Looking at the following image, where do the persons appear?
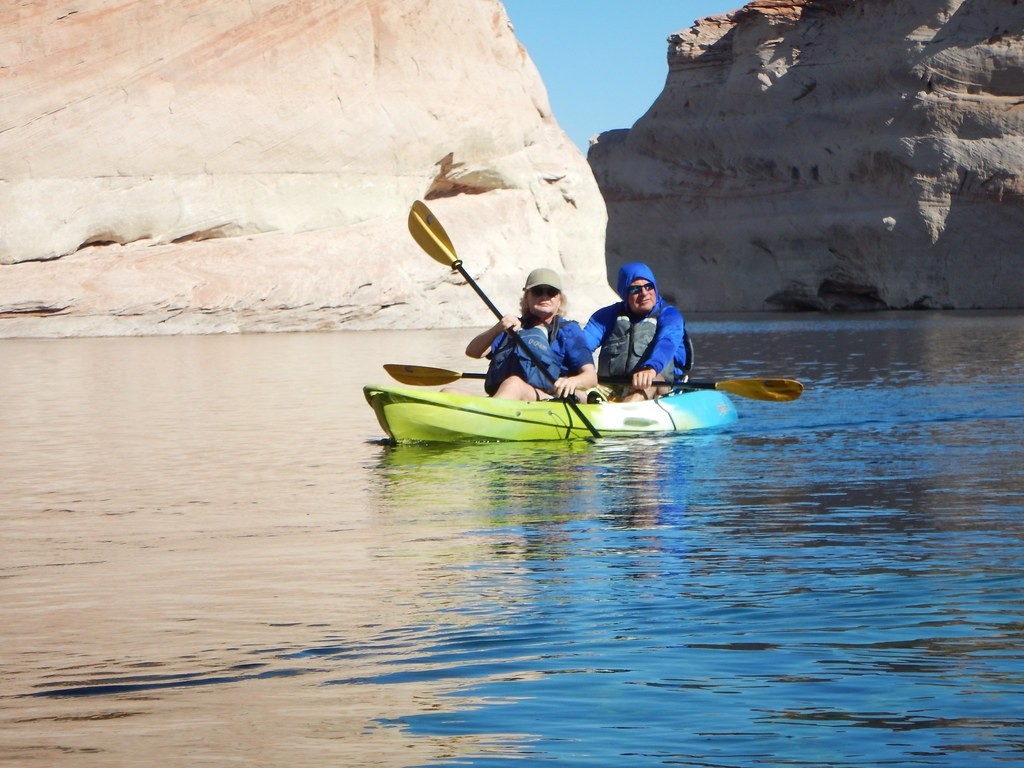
[580,262,688,405]
[466,269,598,402]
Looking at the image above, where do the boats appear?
[363,382,739,443]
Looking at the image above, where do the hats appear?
[524,267,561,295]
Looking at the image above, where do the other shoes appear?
[587,389,607,404]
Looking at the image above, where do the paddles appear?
[407,199,603,441]
[382,357,804,403]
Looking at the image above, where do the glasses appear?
[529,286,558,297]
[629,283,654,293]
